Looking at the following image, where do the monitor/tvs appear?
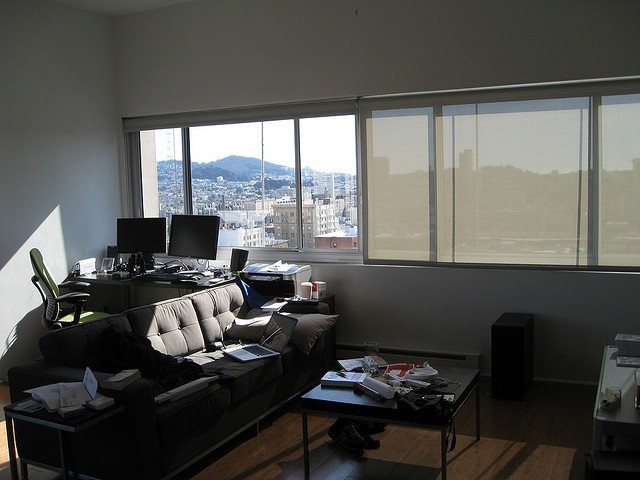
[114,219,167,258]
[167,214,222,273]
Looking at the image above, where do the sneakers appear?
[328,417,366,450]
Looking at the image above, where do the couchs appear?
[7,277,339,477]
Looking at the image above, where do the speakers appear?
[230,248,249,271]
[106,246,118,262]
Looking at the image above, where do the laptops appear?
[223,310,297,364]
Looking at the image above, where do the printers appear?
[240,262,311,300]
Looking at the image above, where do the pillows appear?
[226,307,340,355]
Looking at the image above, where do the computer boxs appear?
[492,312,535,402]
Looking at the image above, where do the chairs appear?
[29,247,119,330]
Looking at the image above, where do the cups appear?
[605,385,621,407]
[300,281,314,300]
[364,340,380,378]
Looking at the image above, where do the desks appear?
[591,344,639,452]
[307,292,337,317]
[72,262,240,309]
[3,393,124,479]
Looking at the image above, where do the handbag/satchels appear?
[397,387,444,420]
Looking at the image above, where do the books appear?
[42,366,114,417]
[610,333,640,367]
[100,369,141,391]
[320,371,367,387]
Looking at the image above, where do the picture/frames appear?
[101,257,115,272]
